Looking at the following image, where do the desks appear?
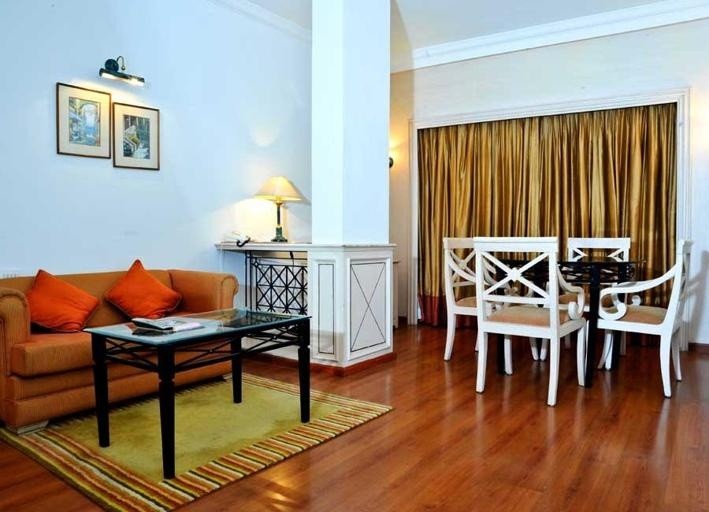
[495,257,646,388]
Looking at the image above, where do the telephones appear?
[221,229,255,248]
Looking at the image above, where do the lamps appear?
[254,176,302,243]
[99,56,145,87]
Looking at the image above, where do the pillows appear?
[103,259,182,320]
[23,269,100,334]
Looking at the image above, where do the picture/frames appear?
[55,82,112,159]
[113,102,161,170]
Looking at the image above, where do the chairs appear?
[564,237,632,355]
[597,238,694,398]
[442,236,539,375]
[473,236,587,406]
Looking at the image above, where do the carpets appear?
[0,368,395,512]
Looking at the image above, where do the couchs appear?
[0,259,239,436]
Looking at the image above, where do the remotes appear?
[131,316,174,333]
